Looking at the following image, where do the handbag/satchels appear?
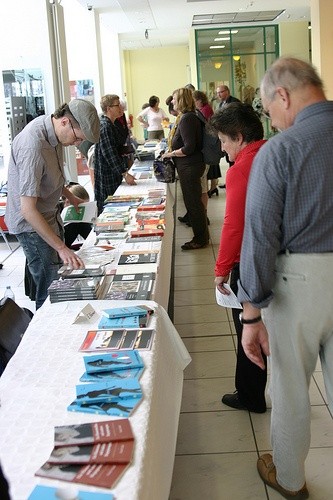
[153,150,175,183]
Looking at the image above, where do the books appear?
[98,314,149,328]
[102,304,154,319]
[78,329,155,350]
[68,351,145,417]
[95,188,166,241]
[132,143,157,178]
[28,419,134,500]
[47,247,157,299]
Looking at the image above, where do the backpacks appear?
[0,297,32,365]
[179,108,224,166]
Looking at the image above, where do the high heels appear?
[207,187,219,198]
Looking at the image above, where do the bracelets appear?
[172,150,176,156]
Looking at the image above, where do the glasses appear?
[69,119,83,143]
[110,103,122,107]
[216,90,227,95]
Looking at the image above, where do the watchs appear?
[239,313,261,324]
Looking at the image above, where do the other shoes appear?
[177,216,191,226]
[219,184,226,189]
[181,239,208,250]
[257,453,309,500]
[222,391,267,413]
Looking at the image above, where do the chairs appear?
[0,297,31,355]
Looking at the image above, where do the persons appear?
[211,102,270,412]
[163,89,210,249]
[216,86,241,188]
[237,58,333,500]
[24,183,89,301]
[166,84,218,225]
[94,93,137,218]
[136,96,169,140]
[5,98,101,309]
[193,91,222,196]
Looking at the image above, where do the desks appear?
[0,143,194,500]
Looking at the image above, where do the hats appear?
[69,98,101,143]
